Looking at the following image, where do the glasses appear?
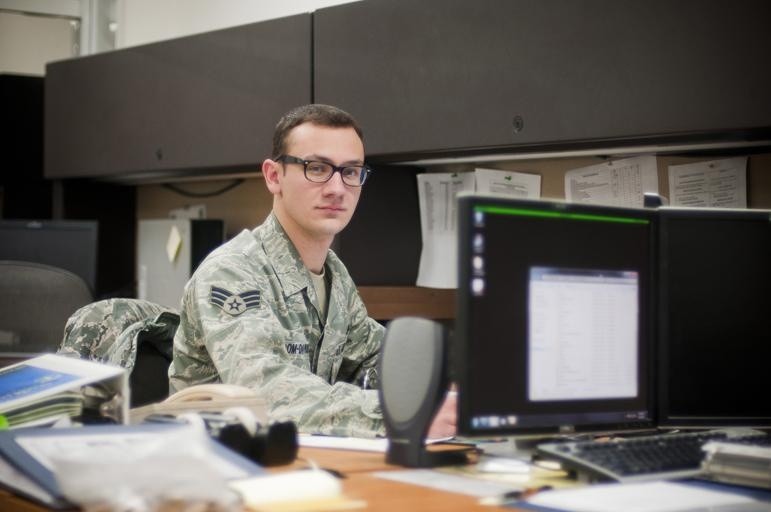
[271,153,371,188]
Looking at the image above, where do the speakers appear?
[377,318,469,467]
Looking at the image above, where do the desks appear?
[0,442,771,512]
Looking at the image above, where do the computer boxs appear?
[137,220,227,313]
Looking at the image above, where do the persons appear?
[166,103,503,442]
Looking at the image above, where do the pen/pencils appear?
[505,484,553,501]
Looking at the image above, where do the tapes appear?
[221,407,260,436]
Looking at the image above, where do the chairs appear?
[0,260,95,352]
[64,296,179,409]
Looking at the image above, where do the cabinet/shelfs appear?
[314,1,770,169]
[43,10,312,179]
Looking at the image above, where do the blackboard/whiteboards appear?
[337,169,438,287]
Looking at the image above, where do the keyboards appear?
[534,432,770,485]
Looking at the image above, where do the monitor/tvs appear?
[658,206,771,425]
[453,191,659,449]
